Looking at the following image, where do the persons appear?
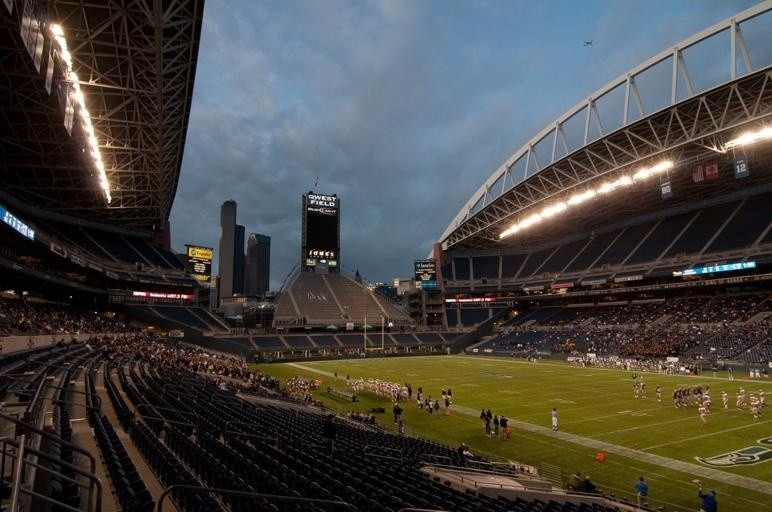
[487,409,493,420]
[494,414,499,437]
[551,407,559,433]
[480,409,487,425]
[484,415,492,438]
[462,446,476,460]
[458,441,466,457]
[630,380,771,429]
[569,471,582,488]
[500,415,509,438]
[634,475,649,507]
[695,480,718,512]
[578,475,597,493]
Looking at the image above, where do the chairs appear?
[272,404,444,511]
[445,445,604,510]
[1,372,270,511]
